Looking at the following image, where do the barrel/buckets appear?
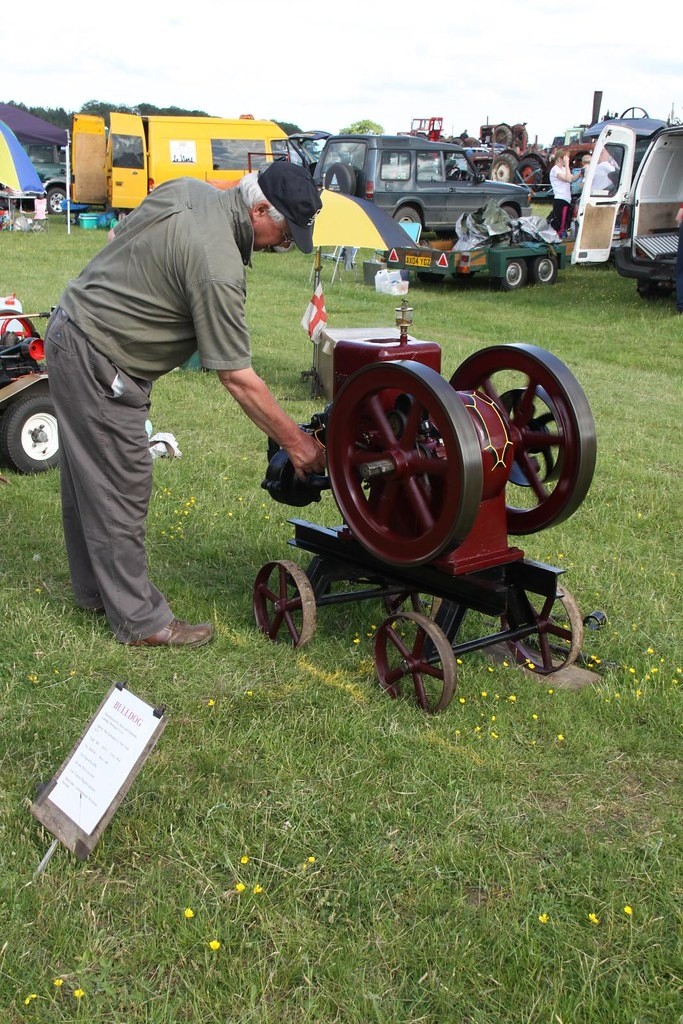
[362,262,381,286]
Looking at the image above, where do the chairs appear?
[21,199,50,234]
[309,245,358,285]
[419,158,440,181]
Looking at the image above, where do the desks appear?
[0,196,37,232]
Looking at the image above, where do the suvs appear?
[312,134,533,238]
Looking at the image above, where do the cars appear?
[21,144,67,214]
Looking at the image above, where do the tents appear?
[0,102,70,234]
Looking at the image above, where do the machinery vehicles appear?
[393,117,597,189]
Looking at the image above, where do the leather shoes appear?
[129,615,213,650]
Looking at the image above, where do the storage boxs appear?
[382,281,410,295]
[78,216,98,229]
[318,327,415,402]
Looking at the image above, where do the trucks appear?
[572,123,683,300]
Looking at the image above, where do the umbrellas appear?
[0,117,47,193]
[314,186,418,332]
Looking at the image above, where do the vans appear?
[446,148,490,171]
[72,112,289,209]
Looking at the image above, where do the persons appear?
[580,148,617,193]
[675,202,683,311]
[460,130,468,142]
[550,149,580,237]
[41,158,327,647]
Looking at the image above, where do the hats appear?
[258,162,325,253]
